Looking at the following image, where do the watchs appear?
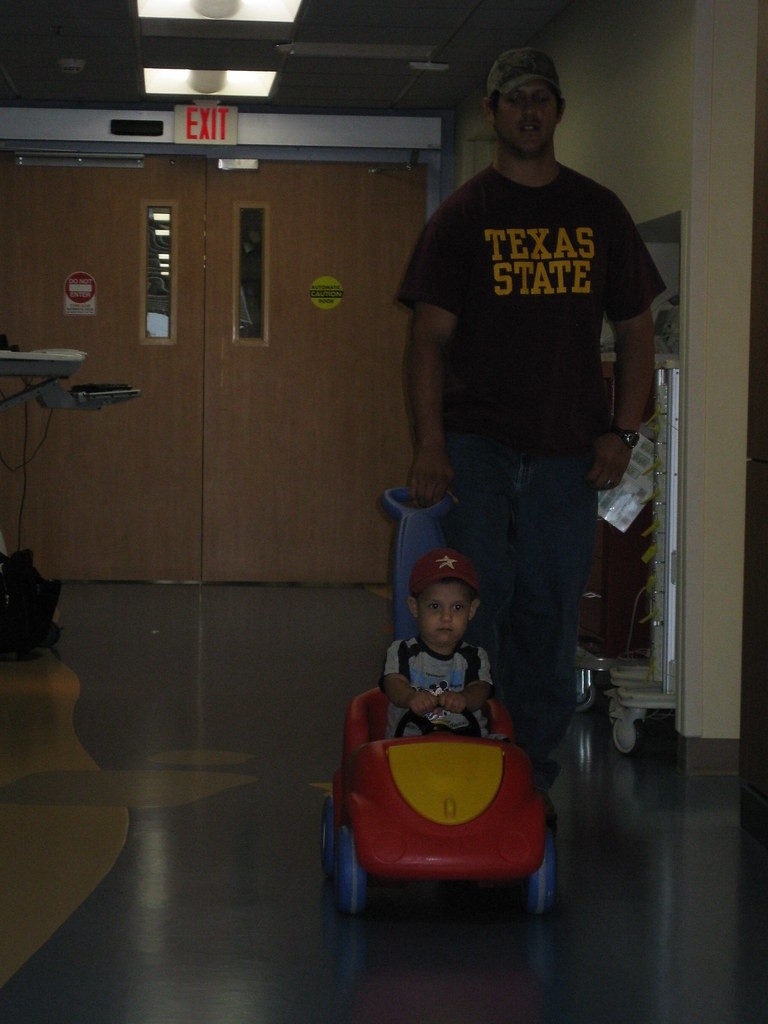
[608,424,640,451]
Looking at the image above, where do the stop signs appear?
[65,270,97,305]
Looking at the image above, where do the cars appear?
[321,688,560,922]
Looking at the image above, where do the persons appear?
[378,547,500,740]
[406,50,656,837]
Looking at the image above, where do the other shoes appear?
[535,788,558,821]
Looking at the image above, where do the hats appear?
[487,47,561,102]
[408,548,481,592]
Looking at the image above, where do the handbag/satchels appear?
[0,548,63,659]
